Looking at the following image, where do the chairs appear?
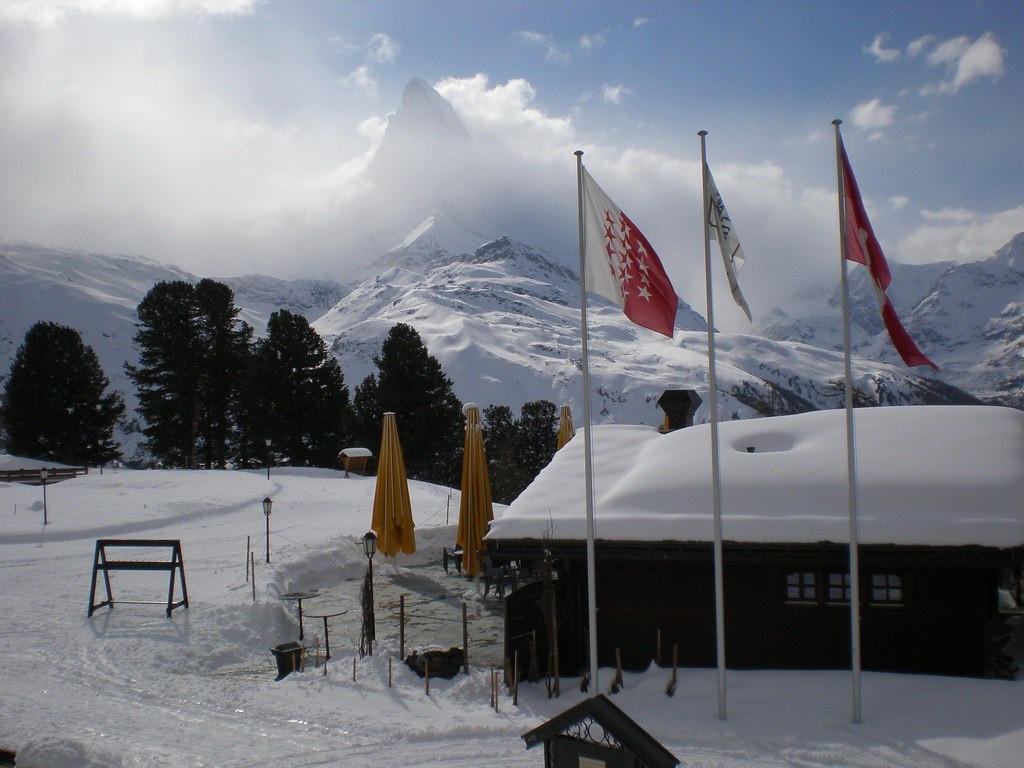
[443,543,464,576]
[475,550,518,601]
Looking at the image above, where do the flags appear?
[581,165,678,340]
[706,163,752,324]
[839,133,941,373]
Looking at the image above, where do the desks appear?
[303,607,348,660]
[279,589,321,641]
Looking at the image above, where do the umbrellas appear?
[456,405,494,592]
[371,411,416,568]
[558,404,576,451]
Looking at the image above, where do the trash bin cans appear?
[268,641,303,682]
